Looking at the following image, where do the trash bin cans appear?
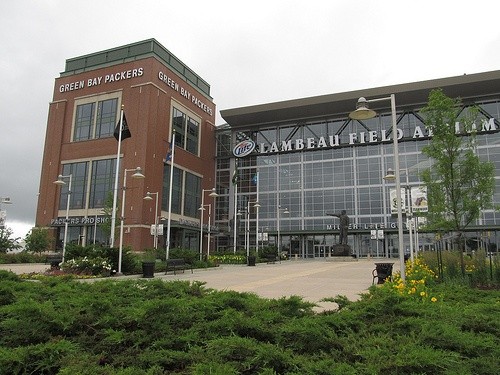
[247,256,256,265]
[374,262,394,284]
[141,260,156,278]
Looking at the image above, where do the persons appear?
[326,210,349,245]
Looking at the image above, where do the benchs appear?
[165,259,194,275]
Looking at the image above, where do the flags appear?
[113,112,131,142]
[165,140,175,161]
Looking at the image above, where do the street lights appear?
[197,204,211,256]
[246,201,262,261]
[349,94,406,285]
[142,191,158,248]
[276,206,290,255]
[200,187,219,258]
[236,207,246,250]
[52,173,72,263]
